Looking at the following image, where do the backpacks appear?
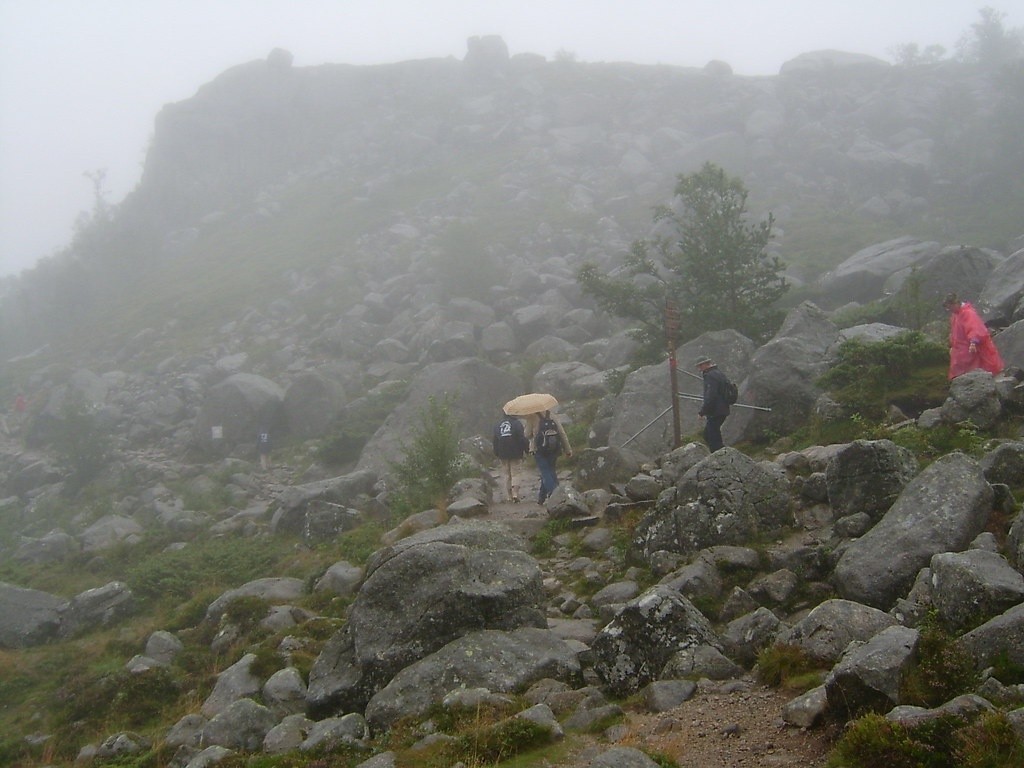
[535,409,563,454]
[710,369,738,404]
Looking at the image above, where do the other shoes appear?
[512,493,520,503]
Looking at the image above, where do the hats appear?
[694,356,711,366]
[943,292,959,306]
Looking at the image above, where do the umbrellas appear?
[502,393,559,416]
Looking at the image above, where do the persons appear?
[493,414,529,502]
[695,354,732,452]
[942,293,1003,380]
[257,434,272,470]
[524,409,573,505]
